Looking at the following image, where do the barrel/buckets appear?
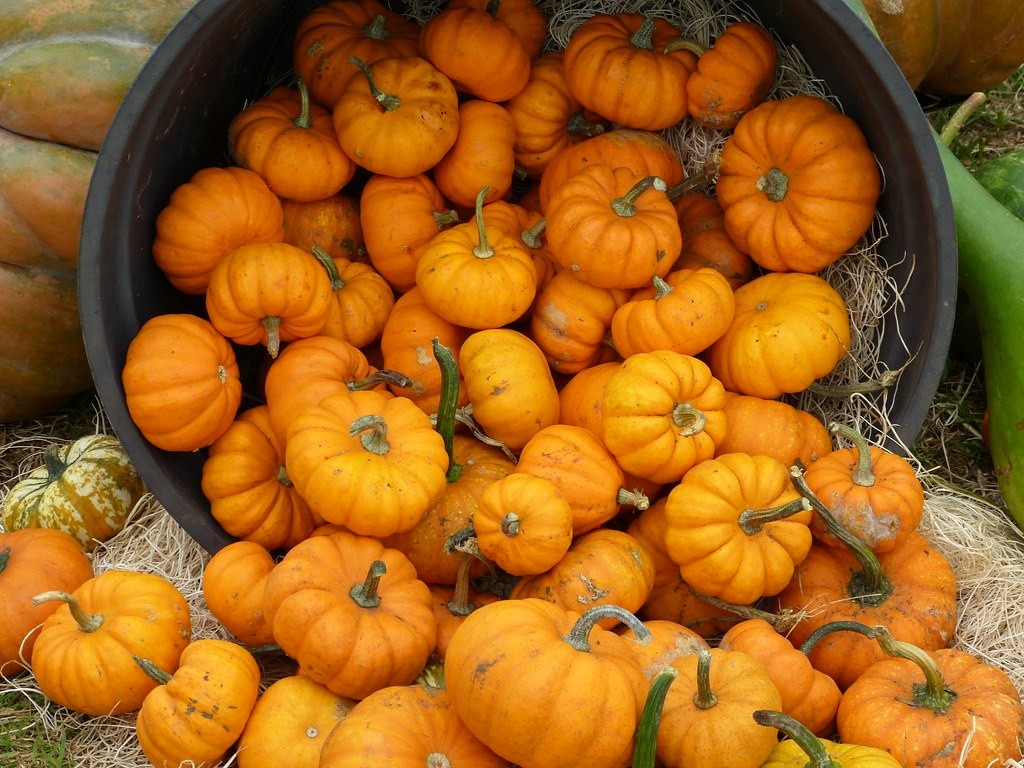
[76,0,956,557]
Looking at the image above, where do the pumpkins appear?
[0,1,1024,768]
